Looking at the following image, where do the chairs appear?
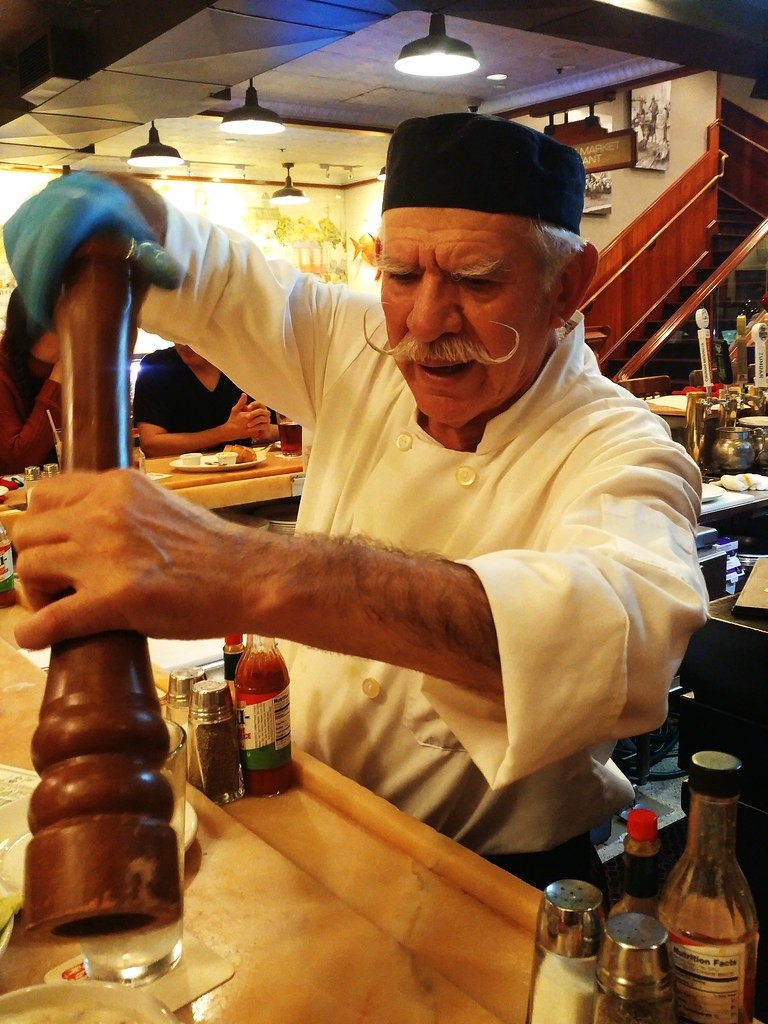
[618,375,672,400]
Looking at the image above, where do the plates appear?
[219,504,300,536]
[0,981,182,1024]
[0,790,198,893]
[169,453,267,472]
[701,483,726,504]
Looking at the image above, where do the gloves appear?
[6,170,183,335]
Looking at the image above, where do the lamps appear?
[270,163,304,198]
[377,167,386,180]
[48,164,72,183]
[221,79,285,134]
[393,13,482,77]
[128,119,184,168]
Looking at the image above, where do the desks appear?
[643,394,752,429]
[695,467,768,525]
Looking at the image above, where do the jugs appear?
[712,427,768,474]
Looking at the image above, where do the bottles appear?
[187,680,246,805]
[166,667,207,735]
[24,466,41,492]
[659,751,759,1024]
[130,428,146,476]
[591,912,677,1024]
[0,521,16,609]
[222,633,246,706]
[604,809,660,917]
[42,464,60,478]
[530,879,604,1023]
[235,632,294,796]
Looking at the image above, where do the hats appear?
[382,113,584,235]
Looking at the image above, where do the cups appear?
[52,429,62,473]
[82,716,188,988]
[216,452,237,466]
[179,453,202,466]
[276,412,302,457]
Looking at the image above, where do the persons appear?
[132,342,280,459]
[0,287,65,477]
[11,114,709,918]
